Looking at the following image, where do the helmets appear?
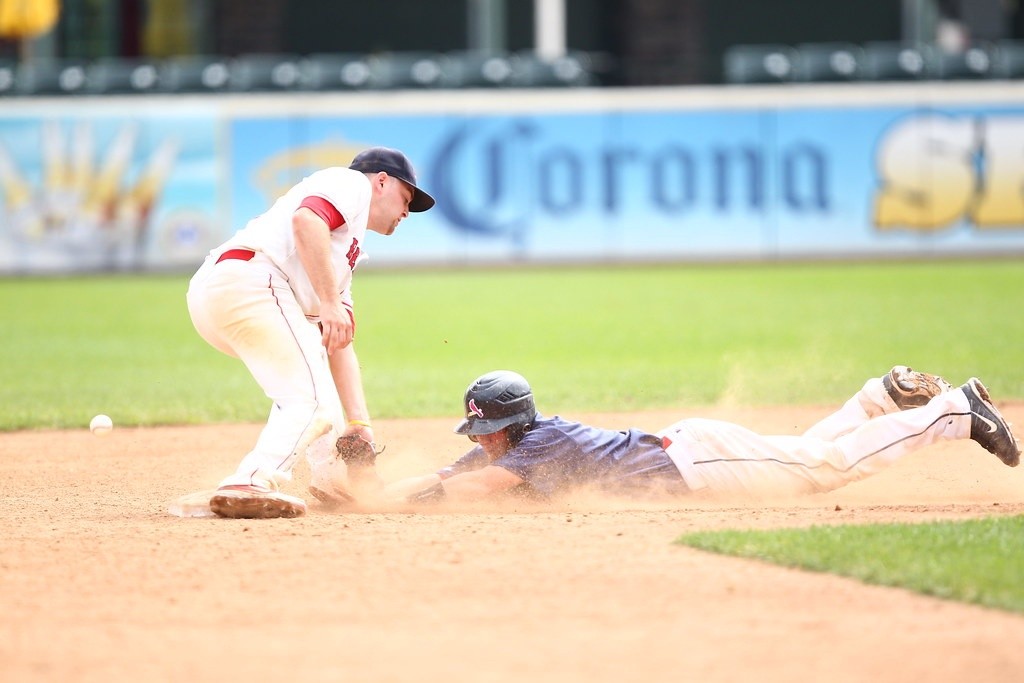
[455,371,540,438]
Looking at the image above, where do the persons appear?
[187,147,435,519]
[309,365,1021,513]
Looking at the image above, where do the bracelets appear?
[348,420,371,427]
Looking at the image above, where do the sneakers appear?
[883,364,954,410]
[961,375,1022,469]
[209,480,306,521]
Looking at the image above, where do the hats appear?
[345,146,435,213]
